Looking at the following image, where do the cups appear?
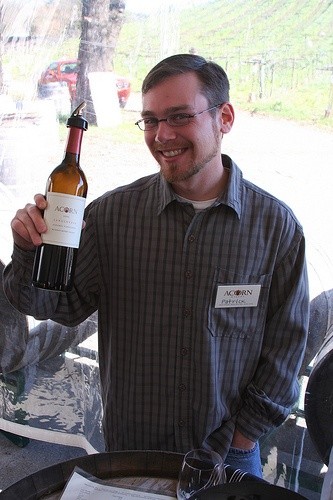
[175,449,226,500]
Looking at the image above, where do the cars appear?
[36,58,132,109]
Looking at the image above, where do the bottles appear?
[31,103,89,293]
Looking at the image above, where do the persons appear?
[0,261,99,448]
[3,54,310,479]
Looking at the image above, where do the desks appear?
[0,451,309,500]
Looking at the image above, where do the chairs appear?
[0,319,98,449]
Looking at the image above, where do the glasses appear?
[135,103,223,131]
